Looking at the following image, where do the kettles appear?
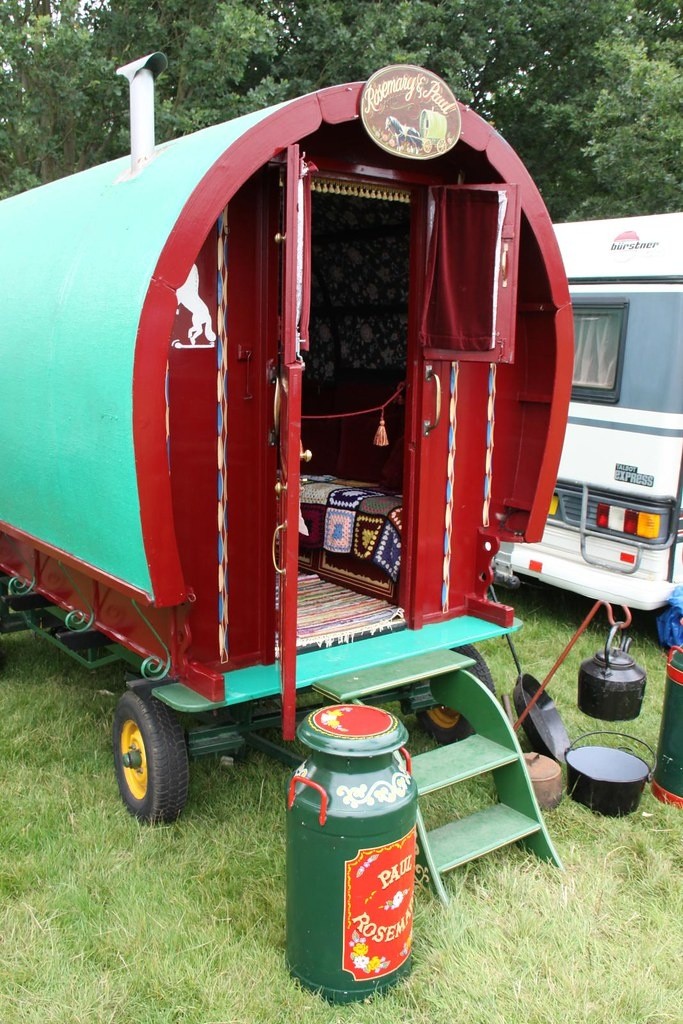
[579,622,647,721]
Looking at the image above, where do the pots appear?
[506,633,570,763]
[523,752,564,810]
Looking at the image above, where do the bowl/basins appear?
[564,731,657,817]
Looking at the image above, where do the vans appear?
[489,210,683,612]
